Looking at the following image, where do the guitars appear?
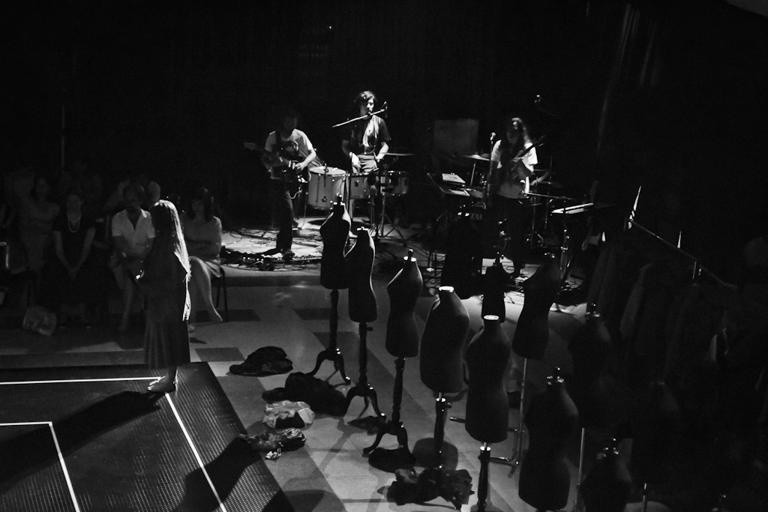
[491,134,545,191]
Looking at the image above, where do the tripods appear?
[426,209,445,278]
[375,195,409,250]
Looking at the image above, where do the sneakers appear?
[282,250,296,262]
[146,375,176,393]
[207,303,222,322]
[115,318,131,332]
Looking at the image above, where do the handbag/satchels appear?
[22,304,58,336]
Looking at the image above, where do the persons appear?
[1,159,224,339]
[310,193,632,511]
[261,108,318,261]
[484,115,539,281]
[336,89,393,223]
[134,198,191,395]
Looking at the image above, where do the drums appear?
[349,172,377,199]
[377,169,412,197]
[308,167,346,210]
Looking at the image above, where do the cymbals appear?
[386,153,411,157]
[455,155,490,164]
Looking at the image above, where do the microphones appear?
[382,97,391,122]
[529,133,549,149]
